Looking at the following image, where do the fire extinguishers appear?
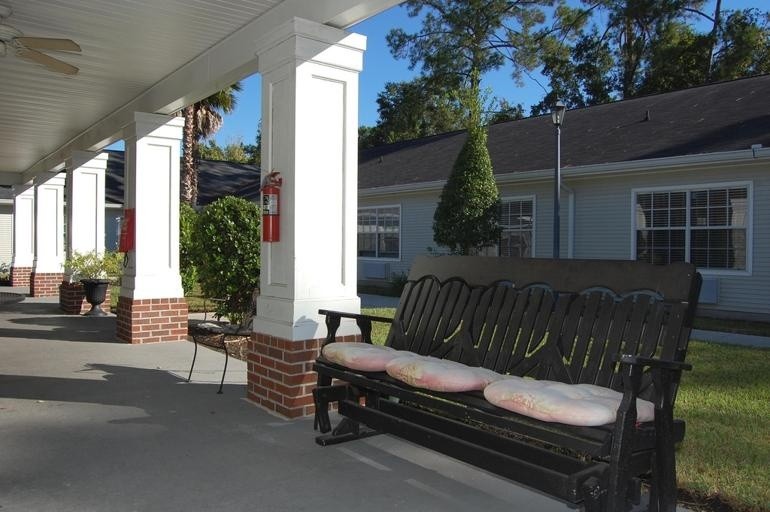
[118,217,128,254]
[259,172,282,242]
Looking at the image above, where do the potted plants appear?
[61,250,121,316]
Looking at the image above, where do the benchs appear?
[311,253,704,512]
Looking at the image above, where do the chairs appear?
[186,283,259,395]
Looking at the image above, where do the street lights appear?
[549,92,568,258]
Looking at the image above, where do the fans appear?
[0,3,83,77]
